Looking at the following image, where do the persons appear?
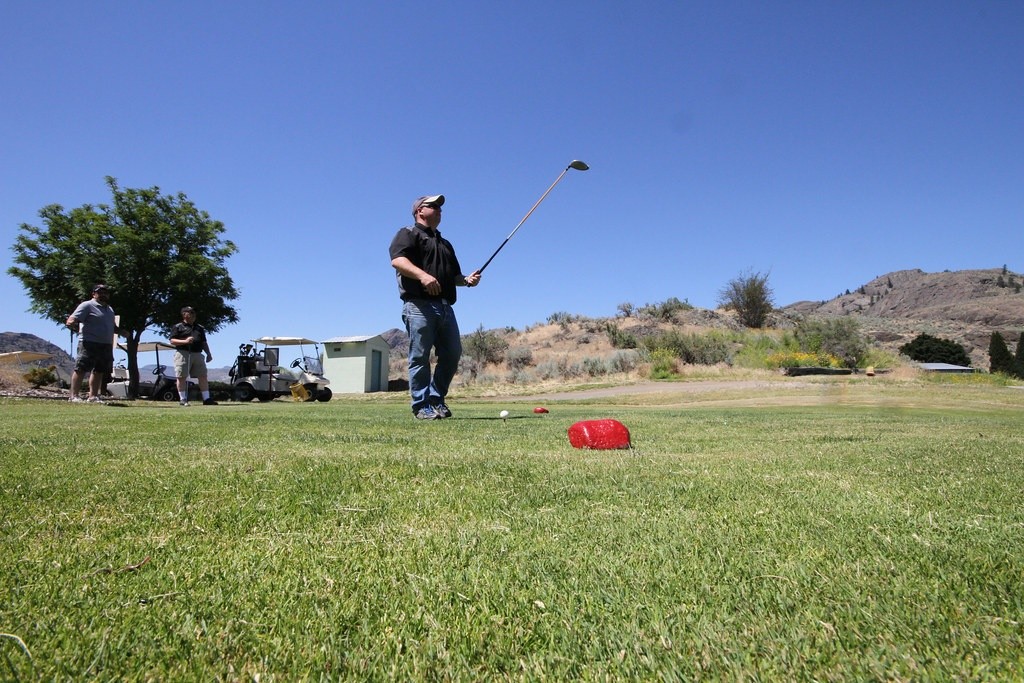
[66,284,115,404]
[169,306,219,407]
[388,195,482,421]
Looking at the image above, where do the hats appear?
[411,194,445,215]
[181,306,194,312]
[92,284,108,292]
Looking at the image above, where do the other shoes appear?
[68,396,85,403]
[202,398,217,405]
[180,399,188,406]
[87,397,106,404]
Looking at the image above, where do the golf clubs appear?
[465,157,592,289]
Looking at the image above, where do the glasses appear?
[420,204,440,210]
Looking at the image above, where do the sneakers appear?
[416,405,441,421]
[430,402,451,418]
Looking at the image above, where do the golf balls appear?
[499,409,510,420]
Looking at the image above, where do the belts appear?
[404,298,450,306]
